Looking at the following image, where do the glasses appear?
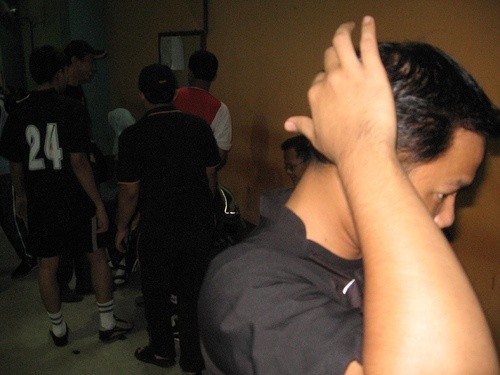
[284,159,306,171]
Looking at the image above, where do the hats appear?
[63,40,108,58]
[138,63,180,93]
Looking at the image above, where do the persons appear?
[115,64,218,375]
[0,69,38,279]
[169,51,235,183]
[56,39,98,306]
[282,133,330,186]
[195,16,500,375]
[0,44,137,348]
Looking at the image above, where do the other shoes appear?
[59,287,83,303]
[134,346,175,368]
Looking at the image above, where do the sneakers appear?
[49,321,69,348]
[11,254,39,280]
[99,314,135,344]
